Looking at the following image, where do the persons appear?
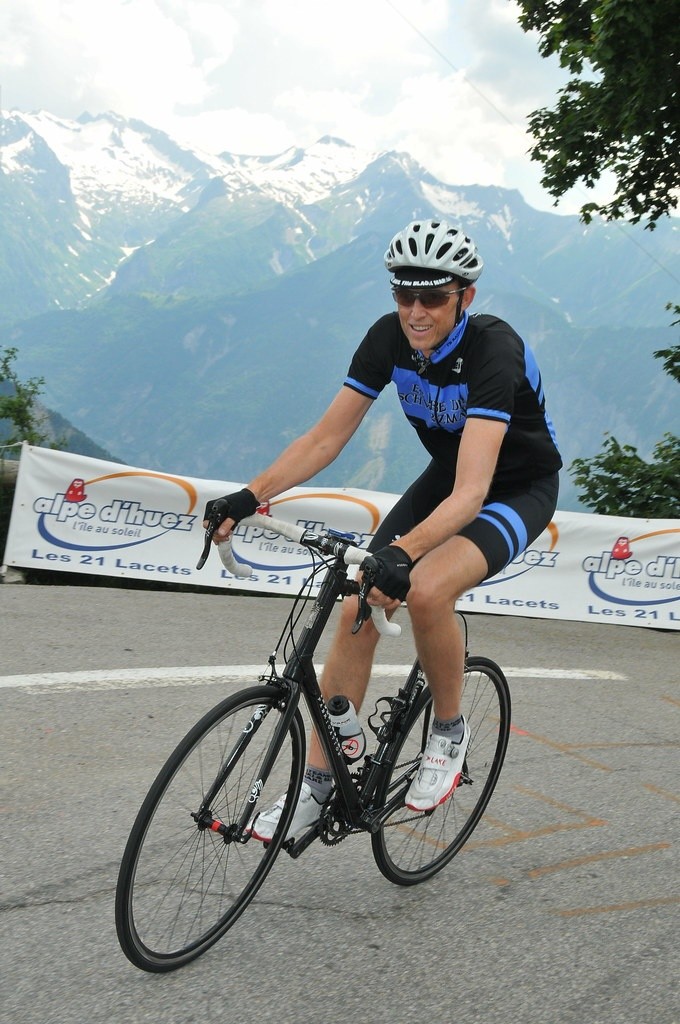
[202,220,562,841]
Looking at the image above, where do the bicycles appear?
[114,499,512,974]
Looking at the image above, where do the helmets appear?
[384,218,484,289]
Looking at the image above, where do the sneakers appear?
[245,777,336,844]
[405,715,471,812]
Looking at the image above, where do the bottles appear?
[327,696,365,759]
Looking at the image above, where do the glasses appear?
[390,285,467,309]
